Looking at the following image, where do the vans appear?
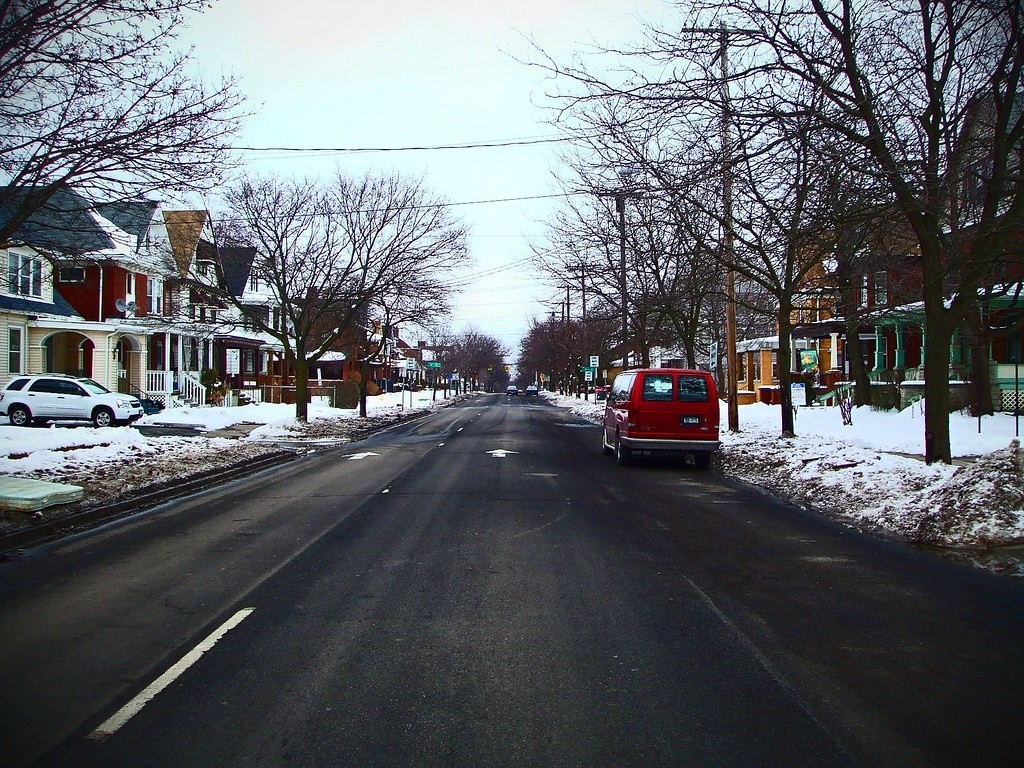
[596,368,721,470]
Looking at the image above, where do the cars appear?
[506,385,518,396]
[526,386,538,395]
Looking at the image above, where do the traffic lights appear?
[505,366,509,372]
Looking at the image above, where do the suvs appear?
[0,373,145,428]
